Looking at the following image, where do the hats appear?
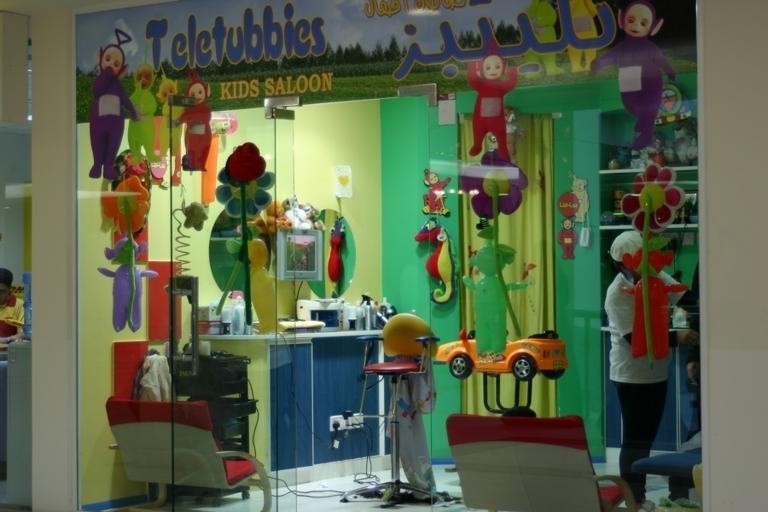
[610,231,642,262]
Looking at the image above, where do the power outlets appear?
[329,412,363,432]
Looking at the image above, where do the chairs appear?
[104,395,275,512]
[348,312,439,507]
[444,411,641,512]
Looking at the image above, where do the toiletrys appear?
[231,296,245,335]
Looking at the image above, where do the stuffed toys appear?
[592,0,678,148]
[97,234,158,332]
[89,27,139,181]
[303,203,326,231]
[155,64,182,157]
[129,43,159,162]
[416,216,453,304]
[422,169,451,215]
[620,246,687,361]
[665,118,698,165]
[328,218,345,281]
[266,201,292,232]
[571,175,589,223]
[557,219,577,258]
[178,70,213,172]
[461,243,528,356]
[282,192,312,231]
[465,38,518,158]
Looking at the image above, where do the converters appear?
[343,409,354,418]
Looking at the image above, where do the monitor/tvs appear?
[277,229,325,282]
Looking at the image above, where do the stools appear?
[631,446,702,502]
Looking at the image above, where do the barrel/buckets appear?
[22,272,32,340]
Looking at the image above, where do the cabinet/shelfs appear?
[602,324,699,478]
[168,351,250,508]
[600,165,698,232]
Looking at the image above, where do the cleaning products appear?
[360,295,373,329]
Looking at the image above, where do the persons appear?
[685,314,700,384]
[0,268,24,361]
[604,230,697,512]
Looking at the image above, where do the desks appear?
[188,327,388,488]
